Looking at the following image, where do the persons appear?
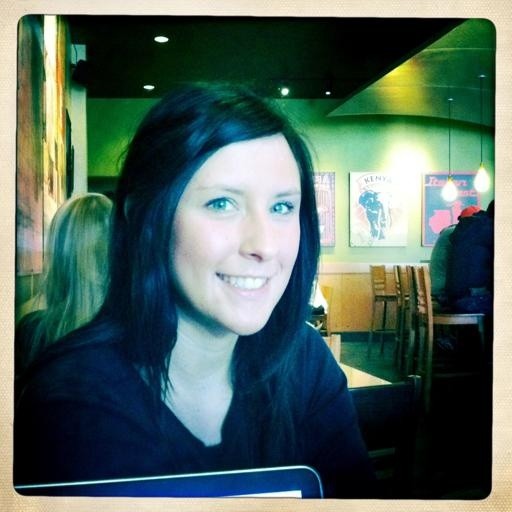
[426,205,482,362]
[446,193,493,350]
[14,76,389,498]
[14,187,117,370]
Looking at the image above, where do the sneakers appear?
[436,335,456,351]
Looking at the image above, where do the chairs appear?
[322,334,341,362]
[367,261,485,408]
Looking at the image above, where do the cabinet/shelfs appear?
[421,170,482,247]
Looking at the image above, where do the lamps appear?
[473,75,491,194]
[441,99,459,203]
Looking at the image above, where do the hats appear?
[461,206,480,218]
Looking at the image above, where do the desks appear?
[336,363,392,389]
